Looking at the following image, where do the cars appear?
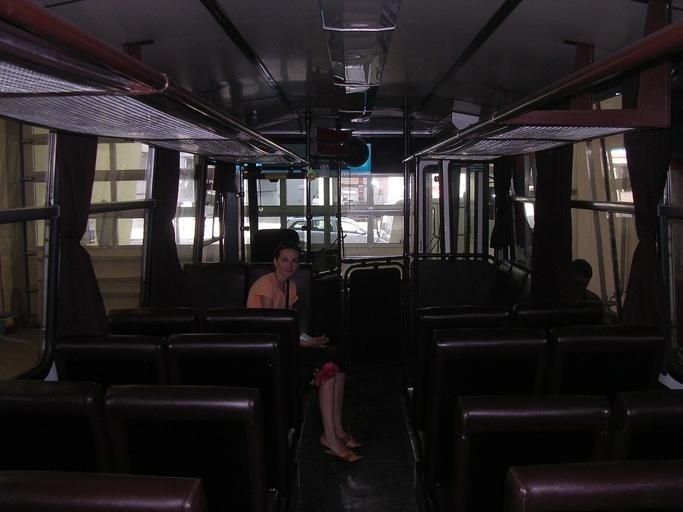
[282,218,387,245]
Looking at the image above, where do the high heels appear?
[339,435,363,448]
[322,449,362,462]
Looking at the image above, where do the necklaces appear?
[274,273,285,294]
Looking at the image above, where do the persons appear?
[246,242,365,461]
